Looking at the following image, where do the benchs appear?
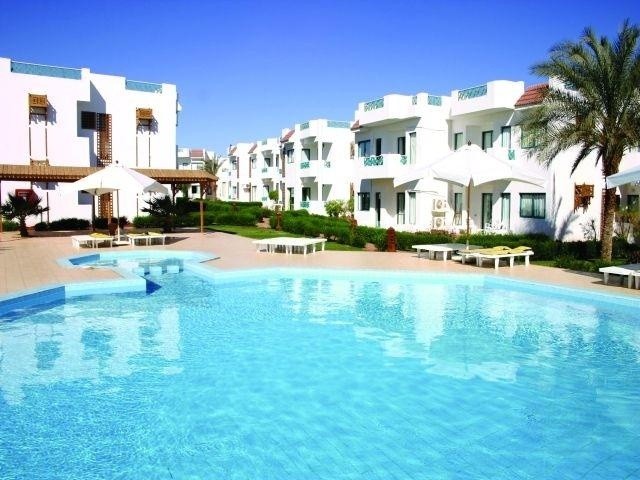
[253,236,327,256]
[596,260,640,291]
[410,242,536,271]
[68,231,166,248]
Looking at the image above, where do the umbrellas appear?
[393,141,545,251]
[69,160,169,242]
[607,165,639,191]
